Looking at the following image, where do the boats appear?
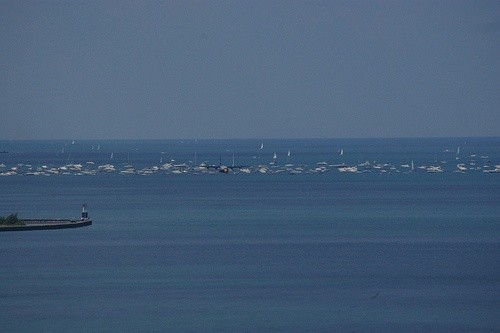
[1,147,498,184]
